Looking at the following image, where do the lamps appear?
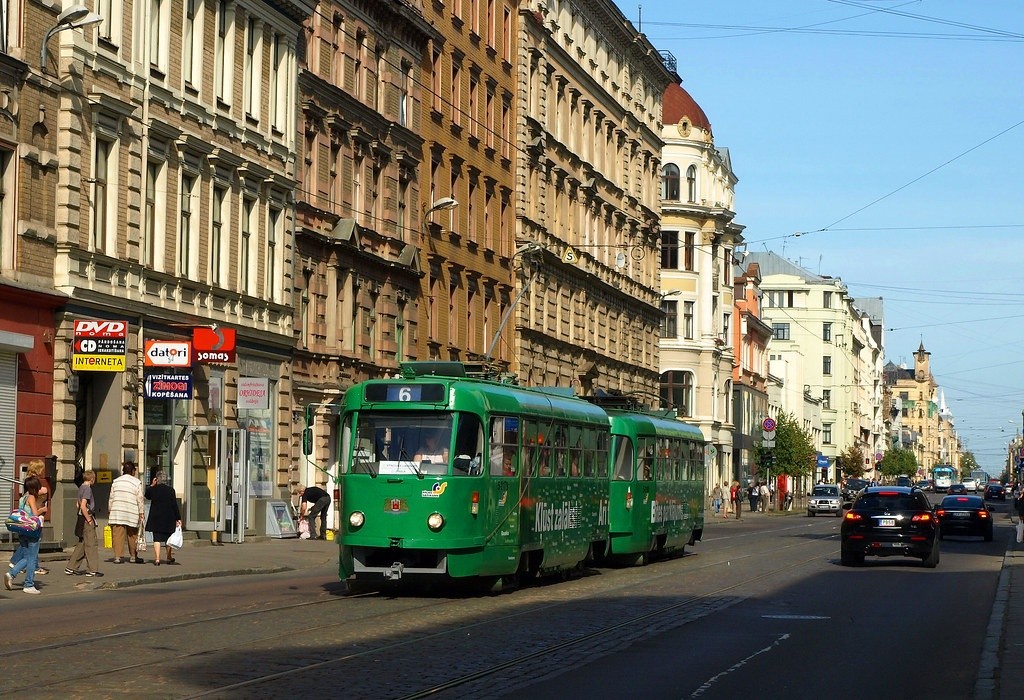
[512,241,541,261]
[424,197,459,221]
[659,291,683,307]
[40,5,104,72]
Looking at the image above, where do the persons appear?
[711,481,773,520]
[108,461,145,564]
[296,484,331,541]
[3,476,47,594]
[65,470,104,577]
[504,454,514,476]
[8,459,49,575]
[412,433,448,465]
[145,471,182,566]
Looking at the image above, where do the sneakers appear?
[23,586,41,594]
[4,573,14,591]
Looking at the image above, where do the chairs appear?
[947,500,955,506]
[830,489,835,494]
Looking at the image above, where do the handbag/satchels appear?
[1016,519,1024,543]
[5,509,42,540]
[75,513,86,538]
[166,524,184,550]
[103,523,113,549]
[136,519,147,551]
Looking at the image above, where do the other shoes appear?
[86,571,104,577]
[154,559,160,566]
[8,562,24,574]
[309,535,317,539]
[129,556,143,563]
[34,567,49,574]
[167,558,175,564]
[113,557,124,564]
[64,567,82,575]
[318,536,326,539]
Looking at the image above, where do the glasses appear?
[133,468,136,471]
[424,436,435,439]
